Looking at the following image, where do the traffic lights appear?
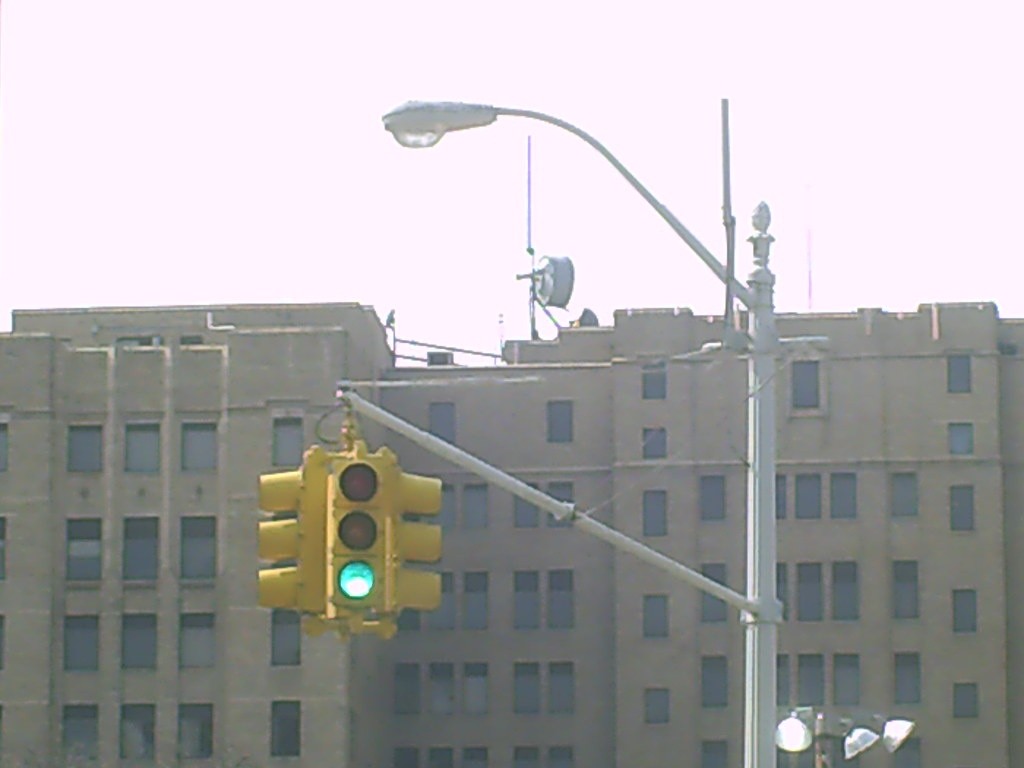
[377,446,442,624]
[255,445,325,622]
[327,452,382,614]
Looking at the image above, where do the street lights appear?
[381,98,781,767]
